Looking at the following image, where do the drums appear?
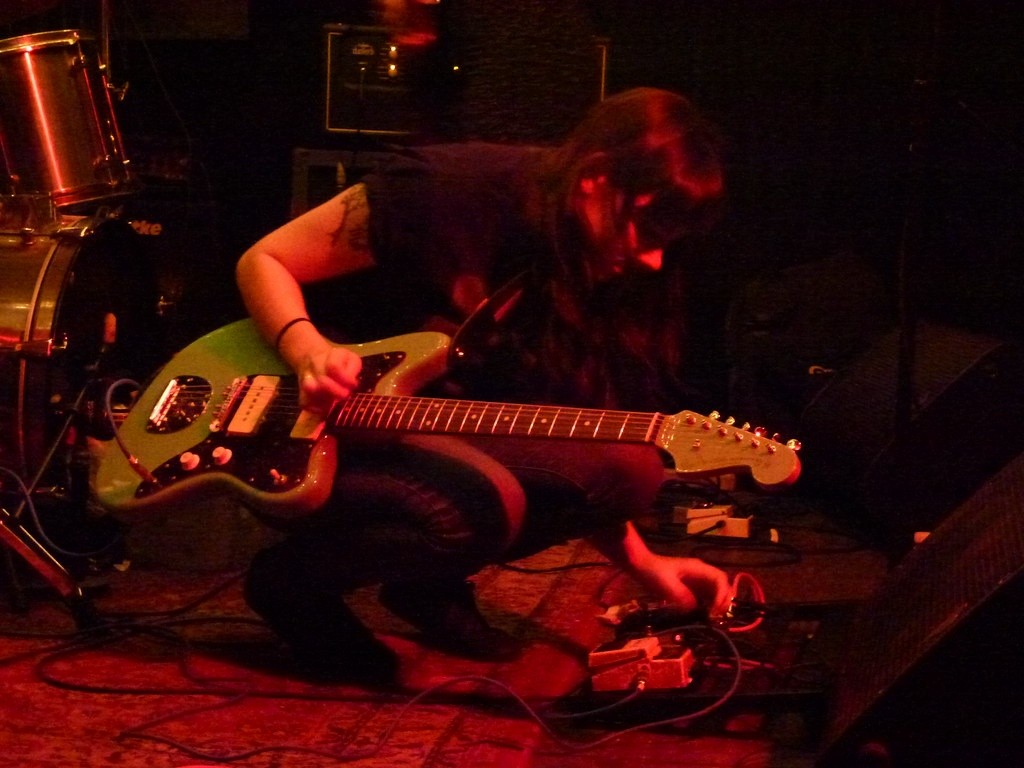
[0,198,112,359]
[0,27,136,211]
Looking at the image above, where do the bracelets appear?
[275,318,313,362]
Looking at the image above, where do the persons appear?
[236,88,734,678]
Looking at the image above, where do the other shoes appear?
[377,576,519,662]
[243,542,398,683]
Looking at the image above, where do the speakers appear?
[795,317,1024,549]
[824,450,1024,768]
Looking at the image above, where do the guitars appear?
[85,311,808,520]
